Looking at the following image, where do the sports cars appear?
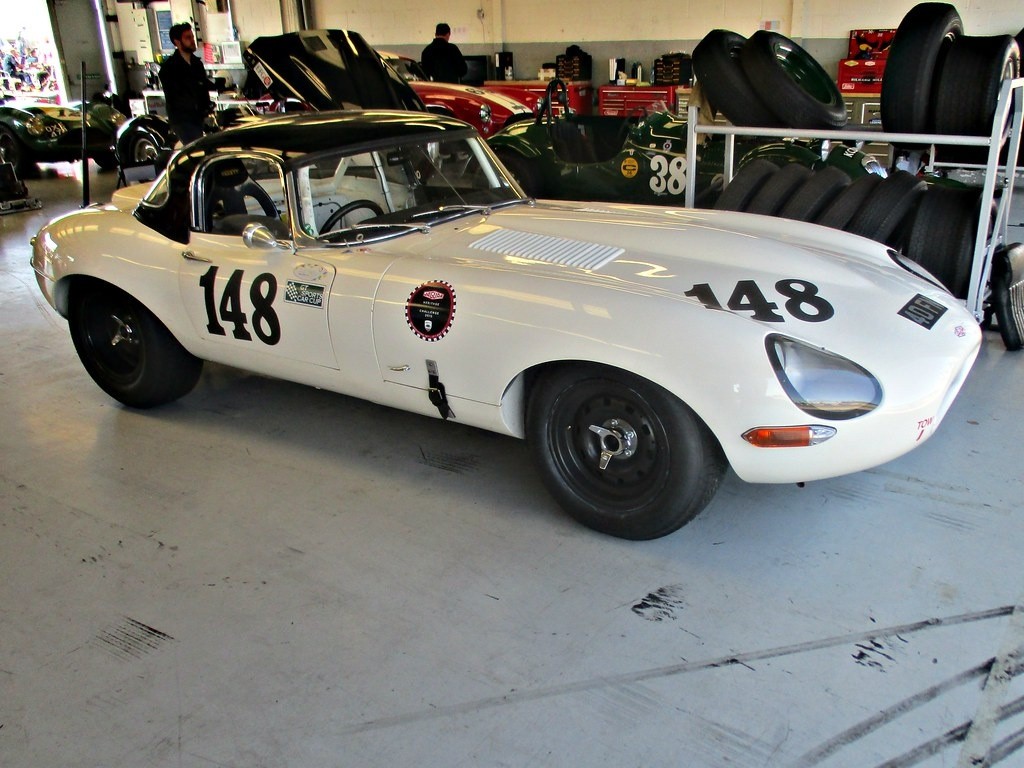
[112,48,544,163]
[31,107,984,540]
[1,98,130,176]
[246,29,890,210]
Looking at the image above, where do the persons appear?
[160,23,216,146]
[421,23,468,84]
[143,61,161,90]
[0,48,50,91]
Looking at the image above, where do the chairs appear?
[197,155,279,233]
